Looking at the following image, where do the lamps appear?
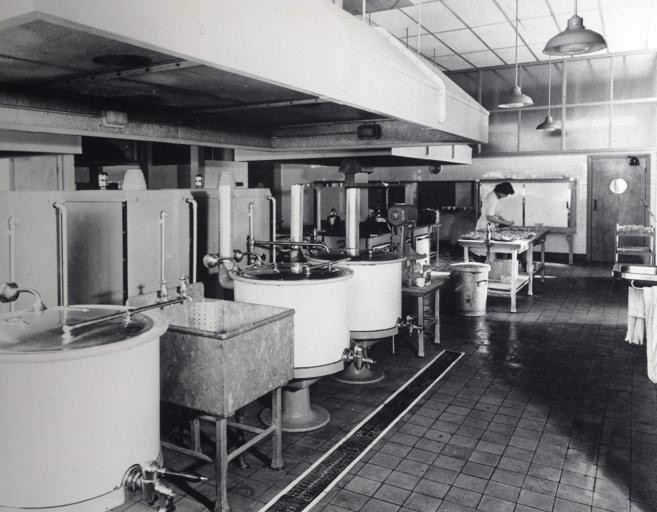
[495,0,607,134]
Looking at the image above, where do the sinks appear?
[127,281,295,419]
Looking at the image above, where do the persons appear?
[469,182,514,262]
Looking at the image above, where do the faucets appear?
[157,281,172,304]
[179,274,193,301]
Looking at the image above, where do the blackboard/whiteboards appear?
[478,177,577,233]
